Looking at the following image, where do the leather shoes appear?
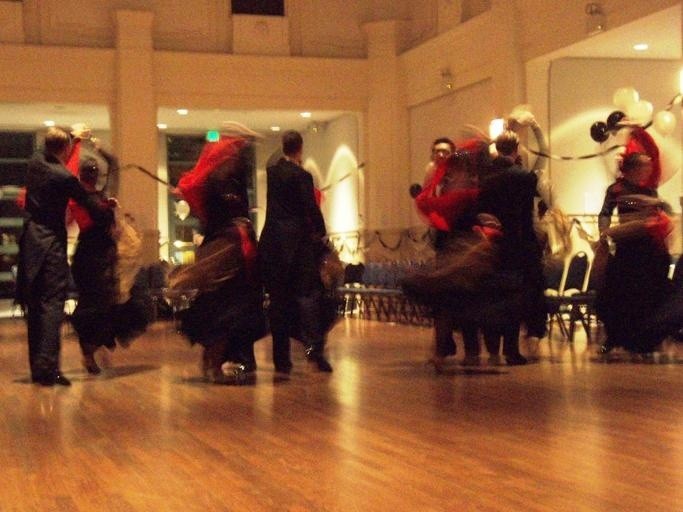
[274,358,293,371]
[505,350,527,364]
[488,355,501,364]
[597,336,614,353]
[461,354,479,365]
[306,344,333,373]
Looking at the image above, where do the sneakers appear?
[31,370,70,386]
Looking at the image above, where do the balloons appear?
[176,200,190,222]
[613,86,638,112]
[628,100,653,127]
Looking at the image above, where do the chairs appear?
[336,259,426,325]
[546,251,600,344]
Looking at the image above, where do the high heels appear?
[235,362,257,374]
[83,358,100,373]
[425,353,456,375]
[208,367,223,383]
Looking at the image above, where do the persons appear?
[11,124,122,386]
[168,123,259,383]
[256,128,344,383]
[398,112,677,365]
[264,154,339,363]
[59,133,163,375]
